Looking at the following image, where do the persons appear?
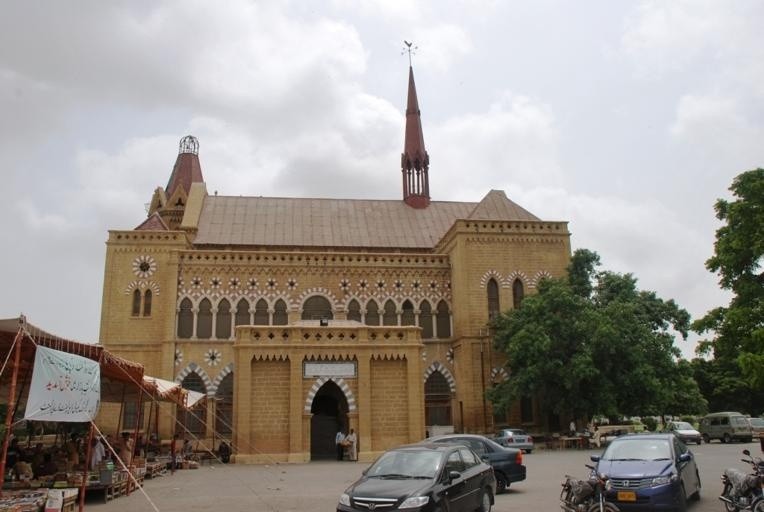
[345,428,357,460]
[589,427,601,449]
[583,426,588,433]
[334,429,346,461]
[643,427,650,432]
[569,420,576,435]
[0,430,232,481]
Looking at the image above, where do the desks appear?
[559,437,582,451]
